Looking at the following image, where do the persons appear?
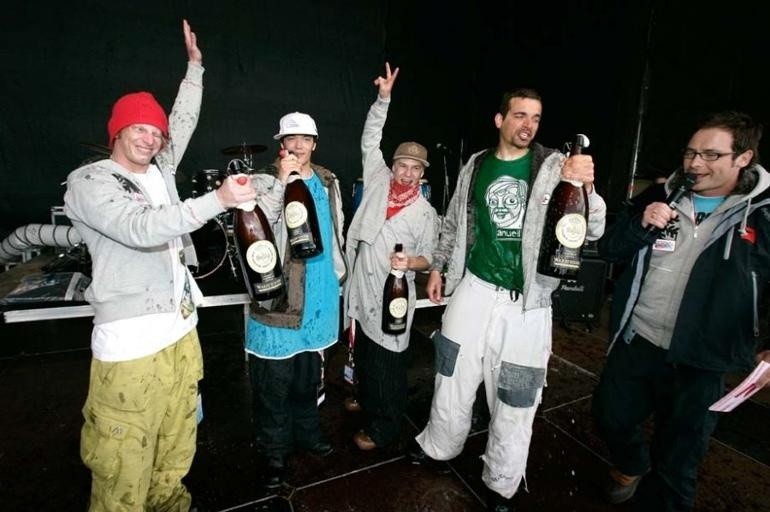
[241,111,350,487]
[592,113,770,512]
[403,89,607,503]
[342,61,441,451]
[62,18,259,512]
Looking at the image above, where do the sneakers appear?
[599,467,643,504]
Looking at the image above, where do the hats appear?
[393,141,430,169]
[107,90,169,150]
[273,110,319,140]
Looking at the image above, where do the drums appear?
[191,169,227,197]
[188,215,229,280]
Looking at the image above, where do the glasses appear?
[682,150,739,161]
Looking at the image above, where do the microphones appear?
[640,171,697,244]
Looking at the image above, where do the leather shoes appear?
[353,430,376,450]
[302,439,334,458]
[345,396,362,413]
[259,468,288,489]
[404,445,437,467]
[490,485,525,512]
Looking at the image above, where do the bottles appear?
[536,139,590,280]
[283,149,325,260]
[381,242,410,337]
[227,159,286,302]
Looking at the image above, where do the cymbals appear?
[223,144,269,155]
